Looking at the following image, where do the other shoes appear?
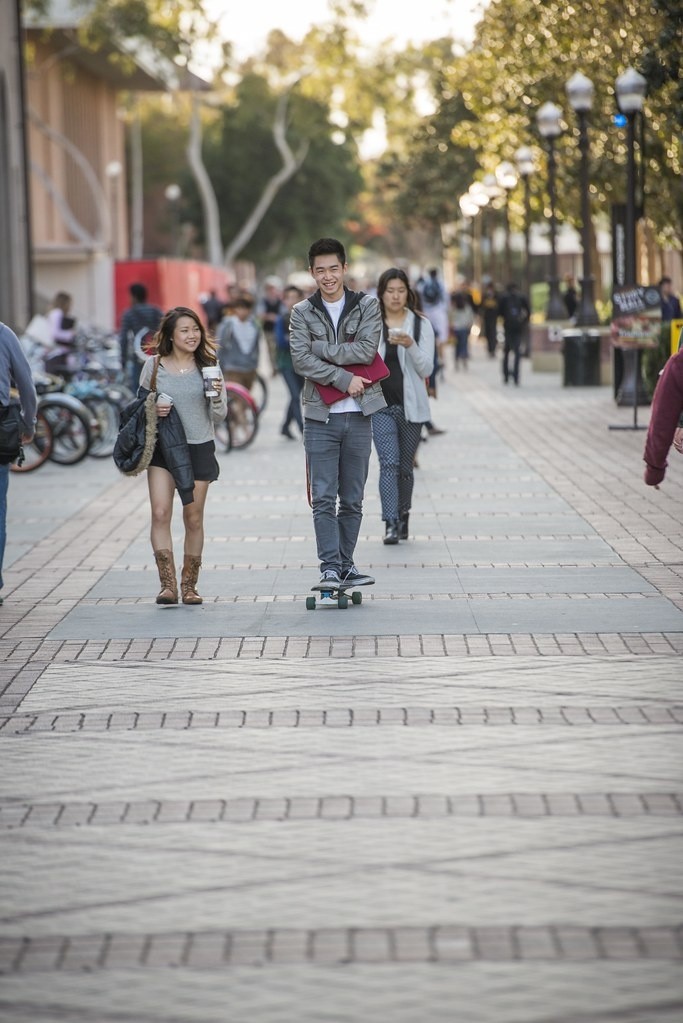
[281,425,296,439]
[395,512,409,540]
[383,519,399,545]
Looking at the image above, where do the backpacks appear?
[423,283,440,304]
[504,298,529,328]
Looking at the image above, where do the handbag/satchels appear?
[0,404,26,468]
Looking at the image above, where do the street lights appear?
[615,66,652,407]
[517,145,537,357]
[537,101,569,320]
[566,68,602,327]
[457,162,519,295]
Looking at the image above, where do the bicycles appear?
[8,329,268,474]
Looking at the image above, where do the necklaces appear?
[167,354,196,374]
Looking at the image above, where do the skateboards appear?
[306,583,364,610]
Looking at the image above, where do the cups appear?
[202,367,220,397]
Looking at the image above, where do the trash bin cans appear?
[562,334,602,386]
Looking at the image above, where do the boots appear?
[180,554,203,604]
[153,549,179,604]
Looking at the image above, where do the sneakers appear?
[319,569,339,584]
[339,564,375,586]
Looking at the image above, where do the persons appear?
[288,237,384,588]
[118,282,164,398]
[371,267,435,546]
[656,276,683,322]
[137,306,230,605]
[0,320,38,608]
[200,265,531,467]
[641,329,683,492]
[561,272,578,319]
[10,290,79,393]
